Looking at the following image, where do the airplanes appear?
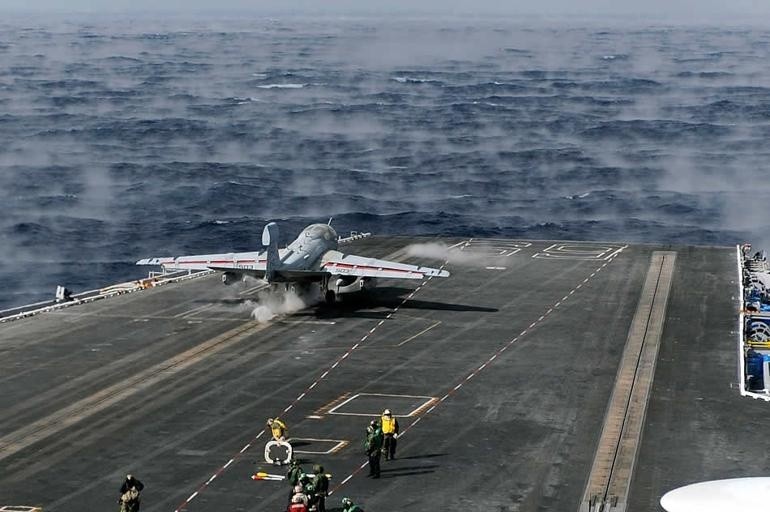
[134,222,450,304]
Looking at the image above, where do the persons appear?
[379,408,399,461]
[117,475,144,512]
[288,459,329,512]
[342,497,363,512]
[266,417,289,441]
[364,420,384,479]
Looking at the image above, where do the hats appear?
[294,486,302,492]
[382,409,391,415]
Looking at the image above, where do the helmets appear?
[126,475,135,484]
[342,498,351,506]
